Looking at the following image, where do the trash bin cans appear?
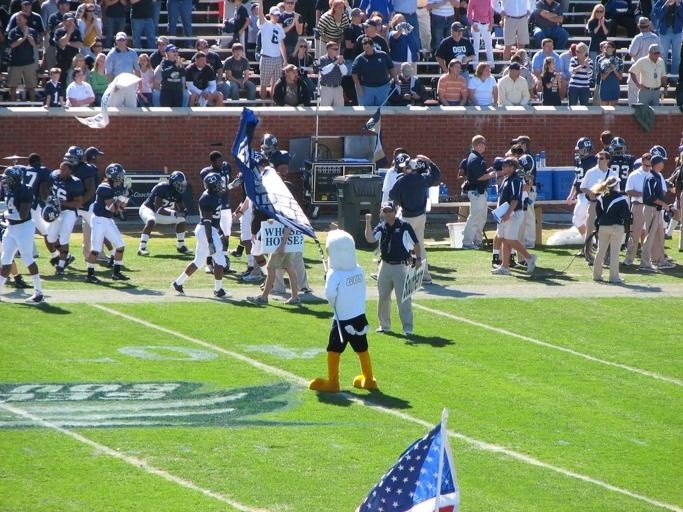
[333,174,382,249]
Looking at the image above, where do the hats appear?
[639,17,650,26]
[84,147,104,159]
[650,154,666,166]
[270,6,282,17]
[56,0,74,21]
[352,7,376,26]
[451,21,465,32]
[571,44,576,56]
[509,62,520,70]
[383,200,395,209]
[494,136,535,175]
[157,36,179,51]
[115,32,128,41]
[606,176,622,188]
[21,0,32,5]
[401,62,413,77]
[649,43,660,53]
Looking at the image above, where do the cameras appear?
[664,210,673,222]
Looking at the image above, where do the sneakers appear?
[50,253,75,275]
[593,263,625,283]
[491,253,537,275]
[15,280,33,288]
[205,265,236,274]
[238,267,311,305]
[371,275,377,280]
[137,248,149,256]
[214,288,231,298]
[576,252,584,257]
[622,255,677,270]
[87,275,100,284]
[664,234,672,240]
[172,282,185,296]
[177,246,193,254]
[423,279,432,285]
[24,294,45,305]
[112,273,130,280]
[462,240,482,249]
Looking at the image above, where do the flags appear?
[354,418,460,512]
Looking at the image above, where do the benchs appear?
[425,200,584,248]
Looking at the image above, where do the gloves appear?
[218,230,226,244]
[174,211,188,217]
[208,238,216,254]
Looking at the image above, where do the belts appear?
[644,87,660,90]
[473,21,485,24]
[508,16,524,19]
[321,84,340,87]
[417,7,423,9]
[386,261,404,264]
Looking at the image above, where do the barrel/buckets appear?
[445,222,468,249]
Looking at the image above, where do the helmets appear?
[611,137,626,156]
[64,146,83,166]
[169,171,188,194]
[650,145,669,161]
[41,204,60,222]
[203,172,222,198]
[254,134,277,163]
[394,147,425,174]
[0,166,24,196]
[575,137,595,160]
[105,163,127,183]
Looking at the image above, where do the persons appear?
[364,200,421,335]
[458,135,538,274]
[308,228,377,392]
[566,130,683,285]
[1,1,683,108]
[1,137,317,305]
[380,148,441,286]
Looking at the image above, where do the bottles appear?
[534,153,541,171]
[540,150,547,169]
[439,182,444,203]
[443,185,448,201]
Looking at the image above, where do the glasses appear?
[300,45,307,48]
[85,10,95,12]
[383,208,393,213]
